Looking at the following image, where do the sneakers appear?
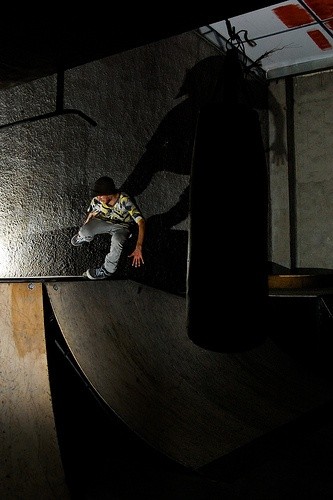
[86,265,113,280]
[71,233,86,246]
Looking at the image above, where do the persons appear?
[70,176,145,279]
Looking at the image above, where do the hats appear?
[94,175,116,195]
